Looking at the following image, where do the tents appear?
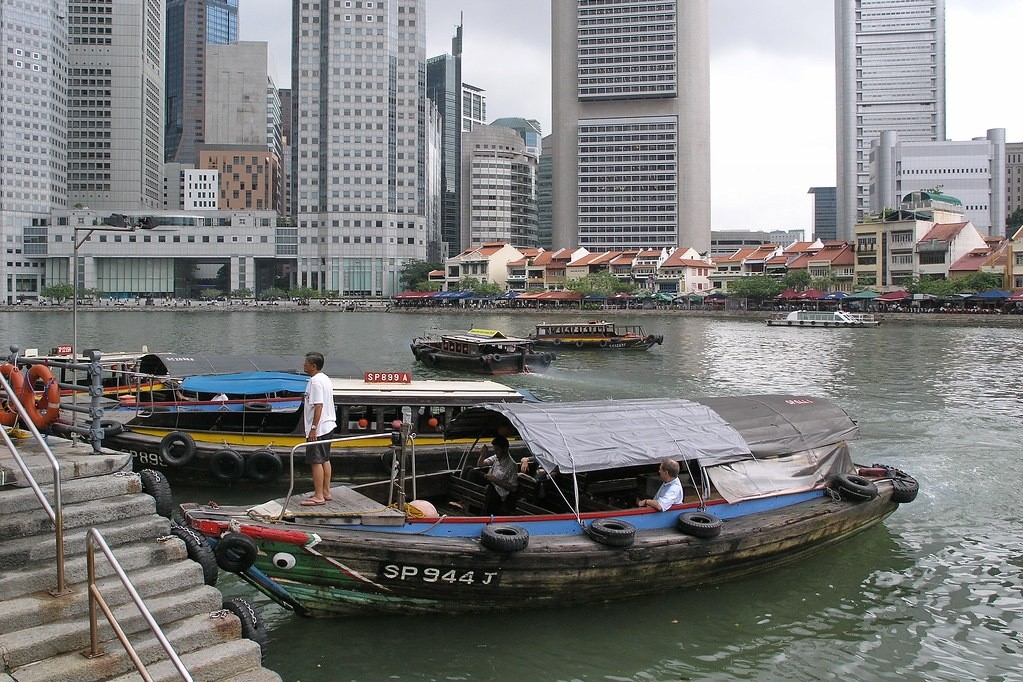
[609,293,738,307]
[394,288,607,308]
[780,288,1023,310]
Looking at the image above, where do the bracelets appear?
[645,499,648,505]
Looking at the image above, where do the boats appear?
[410,323,555,377]
[522,319,665,350]
[1,338,558,492]
[177,392,921,618]
[765,309,881,328]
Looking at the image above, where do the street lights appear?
[71,213,162,450]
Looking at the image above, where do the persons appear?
[639,459,683,513]
[300,352,337,506]
[520,456,534,474]
[477,436,518,516]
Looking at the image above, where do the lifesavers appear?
[208,448,244,479]
[843,322,848,326]
[158,431,196,465]
[799,321,804,324]
[835,322,839,326]
[824,322,828,326]
[0,363,25,426]
[576,341,584,347]
[811,321,815,325]
[589,519,636,547]
[410,343,416,356]
[480,523,529,551]
[21,365,60,427]
[768,319,772,324]
[100,420,121,436]
[647,334,655,342]
[554,339,561,345]
[478,355,486,365]
[851,322,855,327]
[543,354,551,364]
[859,322,864,326]
[171,525,218,587]
[788,321,792,325]
[216,533,258,573]
[246,448,282,482]
[551,352,557,361]
[599,341,607,347]
[493,354,501,362]
[835,472,879,503]
[679,511,723,540]
[415,348,421,362]
[141,468,174,516]
[227,596,262,647]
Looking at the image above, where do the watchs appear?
[311,425,317,430]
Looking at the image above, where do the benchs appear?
[438,465,639,516]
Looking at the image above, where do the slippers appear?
[310,495,333,500]
[301,499,326,506]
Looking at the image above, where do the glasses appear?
[303,361,314,366]
